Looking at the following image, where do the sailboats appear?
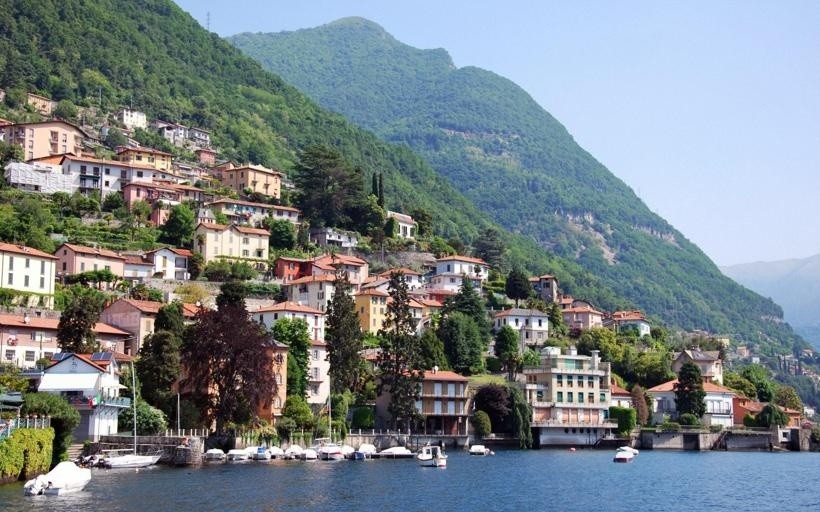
[81,362,165,469]
[316,374,345,460]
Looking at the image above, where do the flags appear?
[89,394,100,408]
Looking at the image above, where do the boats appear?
[200,442,418,465]
[468,443,491,456]
[24,459,94,498]
[416,443,449,468]
[613,446,640,455]
[612,450,634,463]
